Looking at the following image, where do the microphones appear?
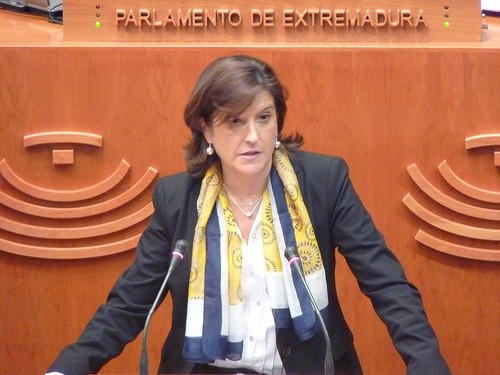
[284,247,335,375]
[139,239,189,375]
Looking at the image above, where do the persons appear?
[42,54,451,374]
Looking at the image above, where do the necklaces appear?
[222,183,263,218]
[225,193,262,206]
